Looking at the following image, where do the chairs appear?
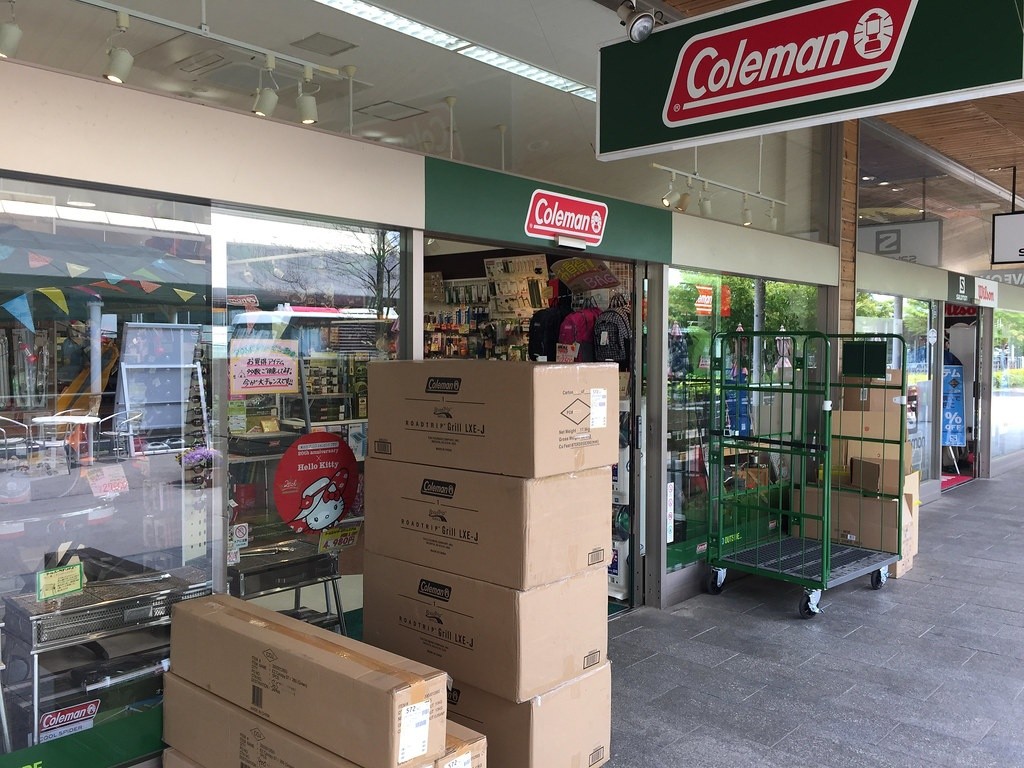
[96,410,145,464]
[0,409,99,477]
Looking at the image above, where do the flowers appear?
[174,445,223,469]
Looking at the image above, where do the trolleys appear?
[703,328,907,621]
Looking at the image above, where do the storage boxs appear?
[162,362,620,768]
[227,432,300,458]
[790,368,919,579]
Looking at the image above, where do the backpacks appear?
[594,295,632,363]
[559,298,602,361]
[528,297,573,362]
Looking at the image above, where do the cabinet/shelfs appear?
[0,565,233,748]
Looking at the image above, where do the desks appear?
[227,454,283,523]
[184,540,348,638]
[31,415,101,466]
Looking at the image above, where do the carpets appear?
[941,474,974,494]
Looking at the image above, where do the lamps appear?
[272,260,284,279]
[696,181,711,218]
[661,172,680,208]
[740,192,752,226]
[295,64,320,125]
[238,261,259,284]
[674,177,694,213]
[617,0,656,43]
[102,12,133,86]
[0,0,22,60]
[249,54,280,118]
[767,201,777,233]
